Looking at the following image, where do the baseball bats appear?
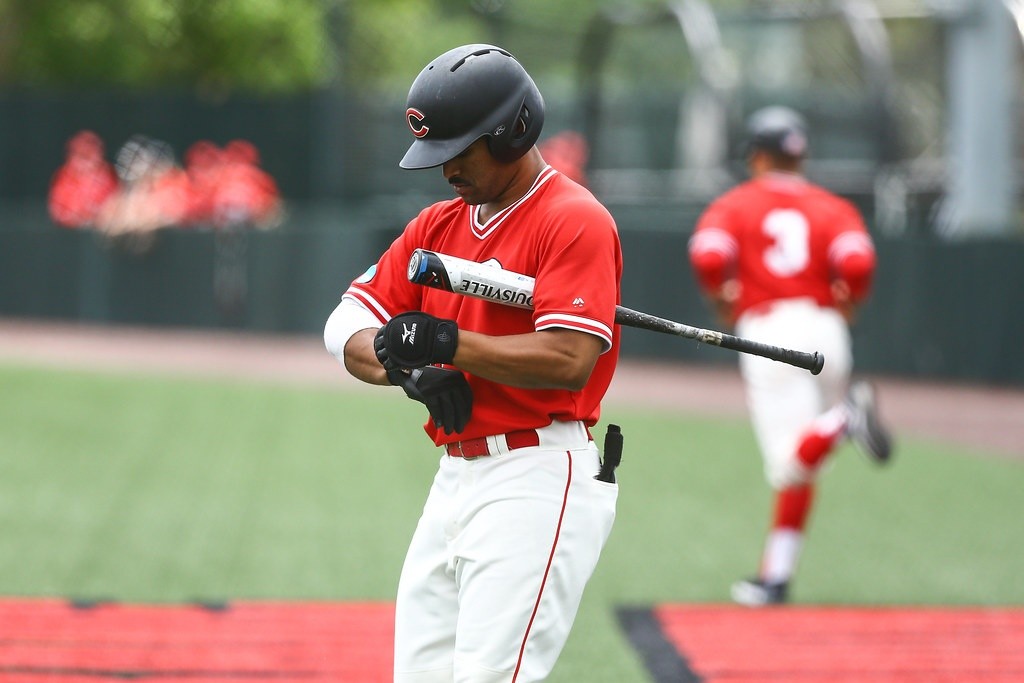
[407,248,825,375]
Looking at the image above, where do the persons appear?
[323,43,624,683]
[688,104,895,608]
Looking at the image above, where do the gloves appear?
[373,312,458,371]
[388,366,474,435]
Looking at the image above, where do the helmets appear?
[731,103,809,159]
[397,44,546,169]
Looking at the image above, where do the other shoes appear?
[846,378,892,466]
[731,577,787,606]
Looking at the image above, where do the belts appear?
[445,425,595,460]
